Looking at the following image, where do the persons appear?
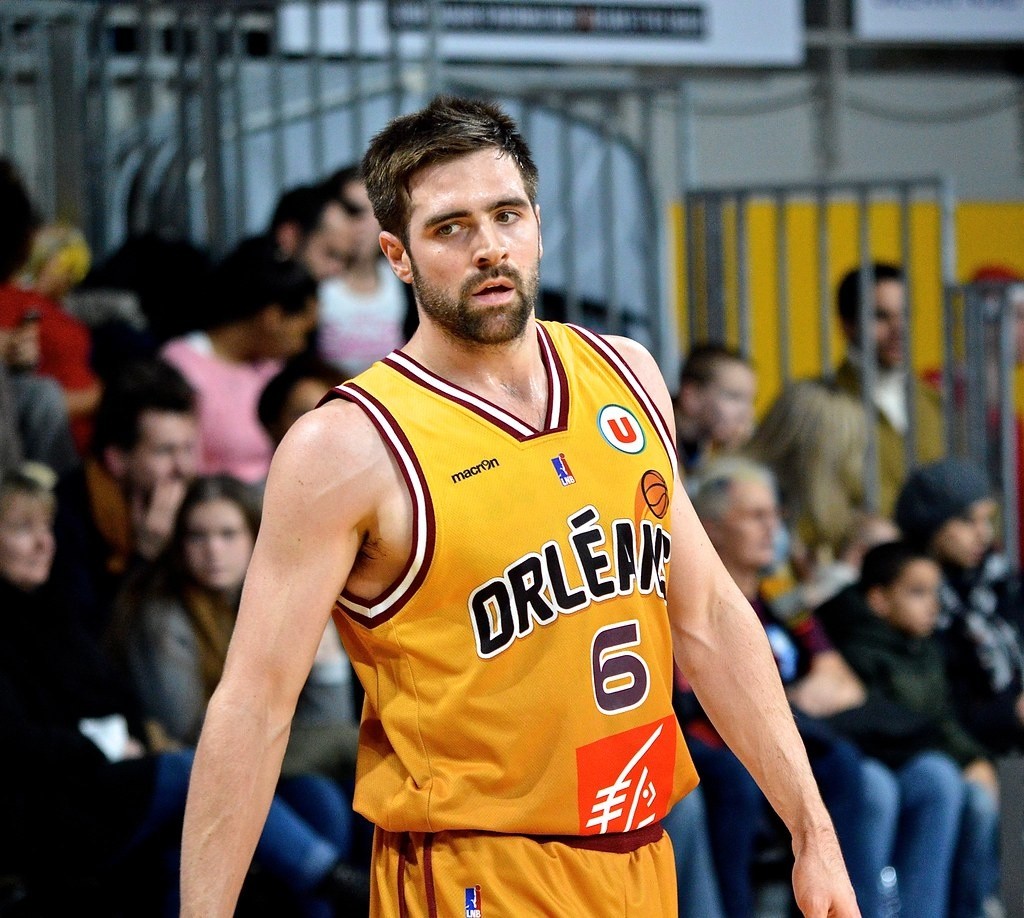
[175,92,864,918]
[0,160,1024,918]
